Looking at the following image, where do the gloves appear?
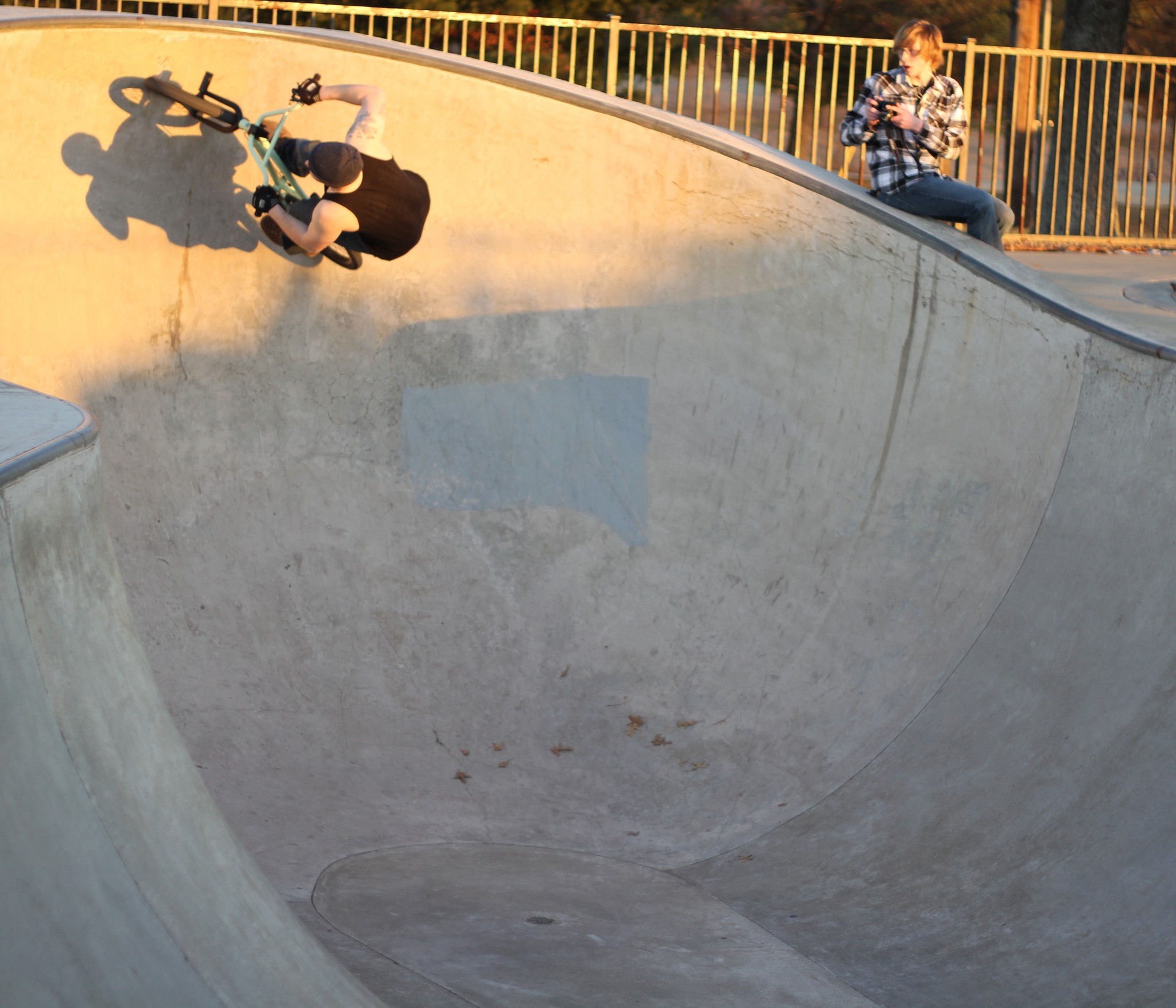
[251,185,279,213]
[292,78,322,105]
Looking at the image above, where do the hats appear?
[308,141,363,187]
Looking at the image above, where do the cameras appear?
[873,100,898,124]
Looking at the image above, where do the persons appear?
[839,20,1015,255]
[250,78,431,262]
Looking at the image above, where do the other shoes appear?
[262,119,290,141]
[260,215,285,246]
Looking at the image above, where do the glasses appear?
[895,47,920,56]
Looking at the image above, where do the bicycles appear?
[143,70,370,275]
